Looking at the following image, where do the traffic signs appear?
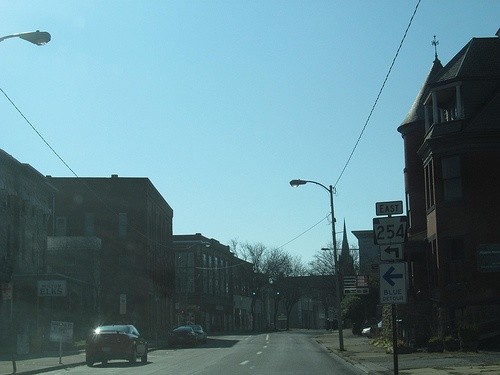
[379,262,407,304]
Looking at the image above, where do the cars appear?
[361,319,402,339]
[84,323,147,367]
[190,324,208,344]
[166,326,198,347]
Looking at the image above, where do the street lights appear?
[289,179,345,352]
